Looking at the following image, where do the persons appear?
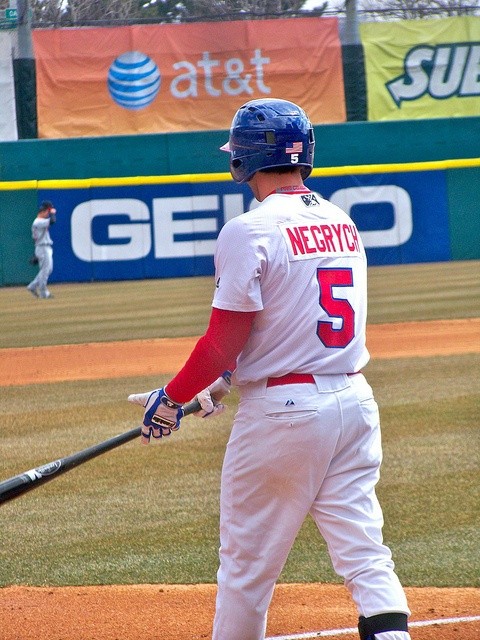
[25,200,57,299]
[127,97,410,640]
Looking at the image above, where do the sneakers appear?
[27,286,40,297]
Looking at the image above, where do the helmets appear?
[219,98,315,186]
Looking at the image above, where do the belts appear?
[266,369,362,388]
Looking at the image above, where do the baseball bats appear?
[0,394,215,506]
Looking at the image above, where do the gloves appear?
[192,369,233,419]
[127,384,185,445]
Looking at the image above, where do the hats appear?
[40,201,53,211]
